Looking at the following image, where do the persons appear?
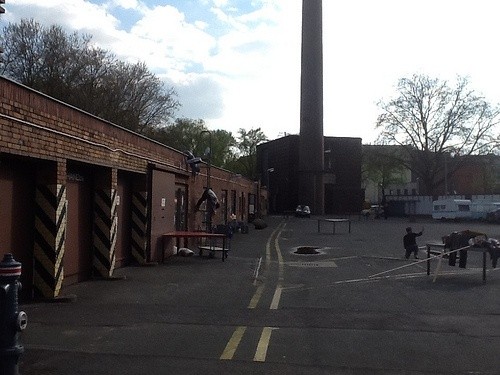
[403,228,424,259]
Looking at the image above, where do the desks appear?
[318,218,351,234]
[426,240,488,283]
[161,231,226,263]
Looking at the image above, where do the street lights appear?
[266,167,276,216]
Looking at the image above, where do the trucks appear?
[430,199,473,223]
